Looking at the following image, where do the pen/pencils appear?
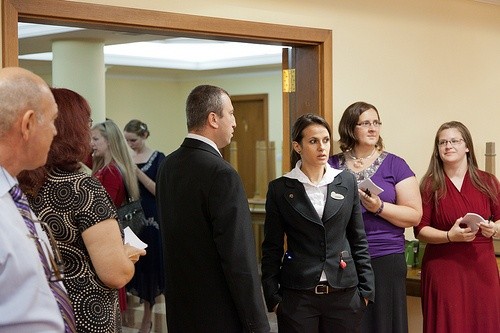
[364,188,371,197]
[487,214,494,224]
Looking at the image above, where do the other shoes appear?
[138,320,153,333]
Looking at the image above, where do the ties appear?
[9,186,77,333]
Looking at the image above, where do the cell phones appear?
[340,251,349,257]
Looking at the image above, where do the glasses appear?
[436,138,464,146]
[27,220,66,282]
[357,121,382,128]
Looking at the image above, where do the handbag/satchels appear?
[114,164,147,240]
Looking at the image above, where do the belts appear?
[307,282,332,295]
